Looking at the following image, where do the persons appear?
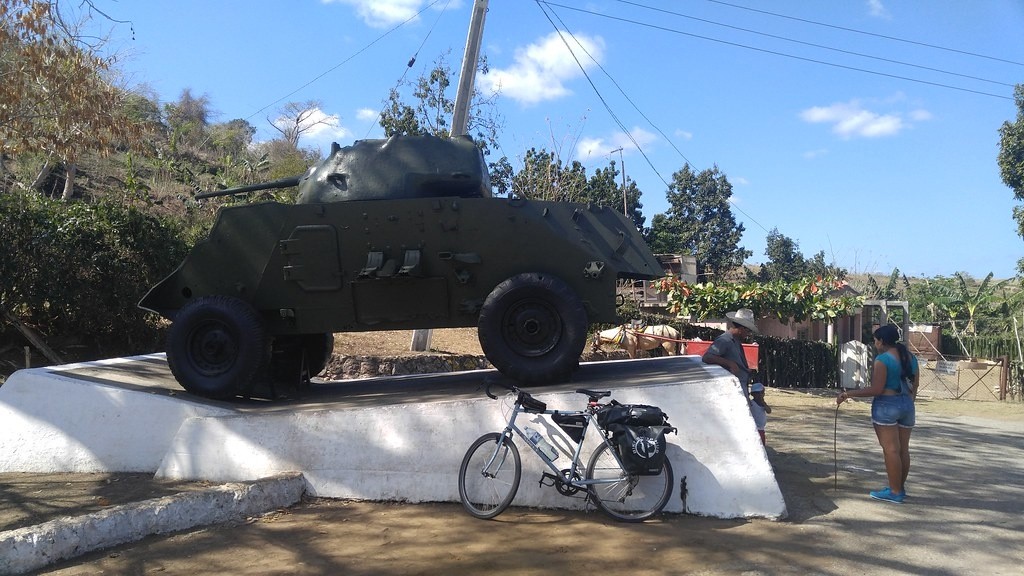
[749,382,771,447]
[703,307,760,408]
[837,326,919,504]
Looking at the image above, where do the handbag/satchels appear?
[597,399,669,430]
[612,423,674,477]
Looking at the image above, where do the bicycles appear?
[458,382,677,523]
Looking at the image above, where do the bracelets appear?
[843,392,848,398]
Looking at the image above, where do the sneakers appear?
[881,486,905,497]
[870,489,903,504]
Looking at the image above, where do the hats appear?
[751,383,764,392]
[725,308,759,333]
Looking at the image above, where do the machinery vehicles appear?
[135,133,668,399]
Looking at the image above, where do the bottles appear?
[524,425,559,462]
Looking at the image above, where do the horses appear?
[590,323,680,359]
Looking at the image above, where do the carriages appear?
[591,318,759,373]
